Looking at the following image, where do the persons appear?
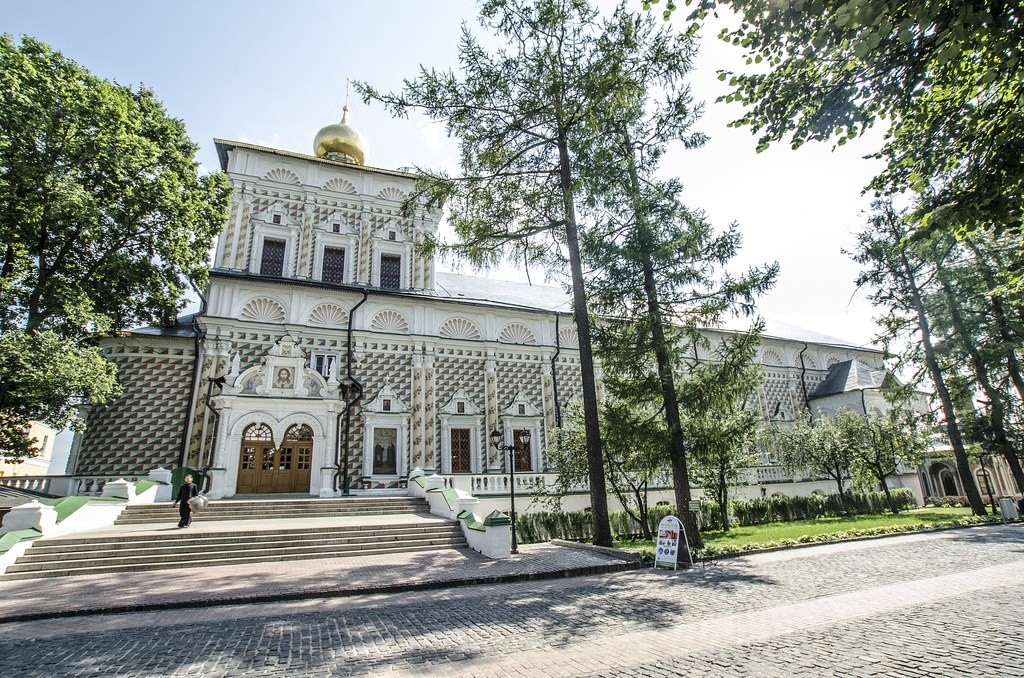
[275,368,292,387]
[383,401,390,410]
[173,475,198,528]
